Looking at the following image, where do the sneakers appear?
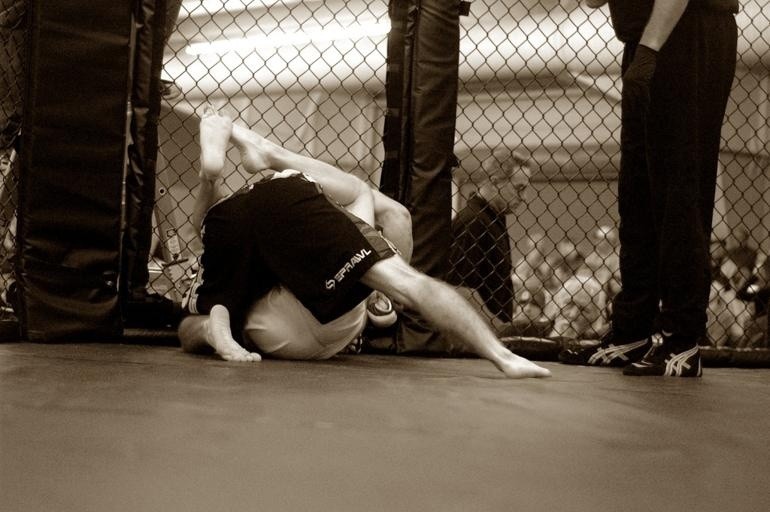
[557,334,652,366]
[622,343,702,376]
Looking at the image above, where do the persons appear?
[192,108,413,360]
[557,0,739,377]
[514,225,769,347]
[176,166,554,378]
[444,150,532,322]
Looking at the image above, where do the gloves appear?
[623,44,658,114]
